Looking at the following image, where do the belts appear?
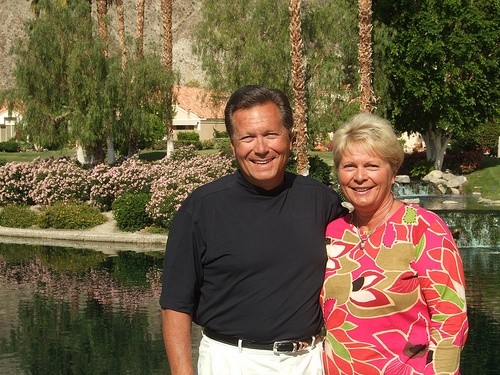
[203,327,321,354]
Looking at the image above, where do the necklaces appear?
[353,191,394,249]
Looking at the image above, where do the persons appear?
[319,112,469,375]
[158,84,350,375]
